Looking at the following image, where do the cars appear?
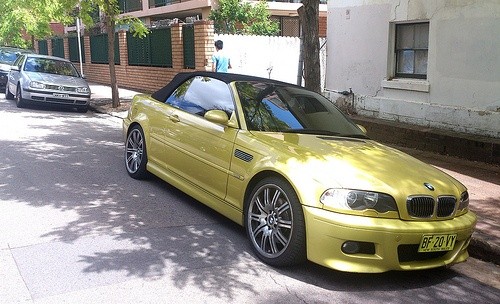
[6,54,91,113]
[121,70,477,275]
[0,46,38,93]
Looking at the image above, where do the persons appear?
[211,40,232,74]
